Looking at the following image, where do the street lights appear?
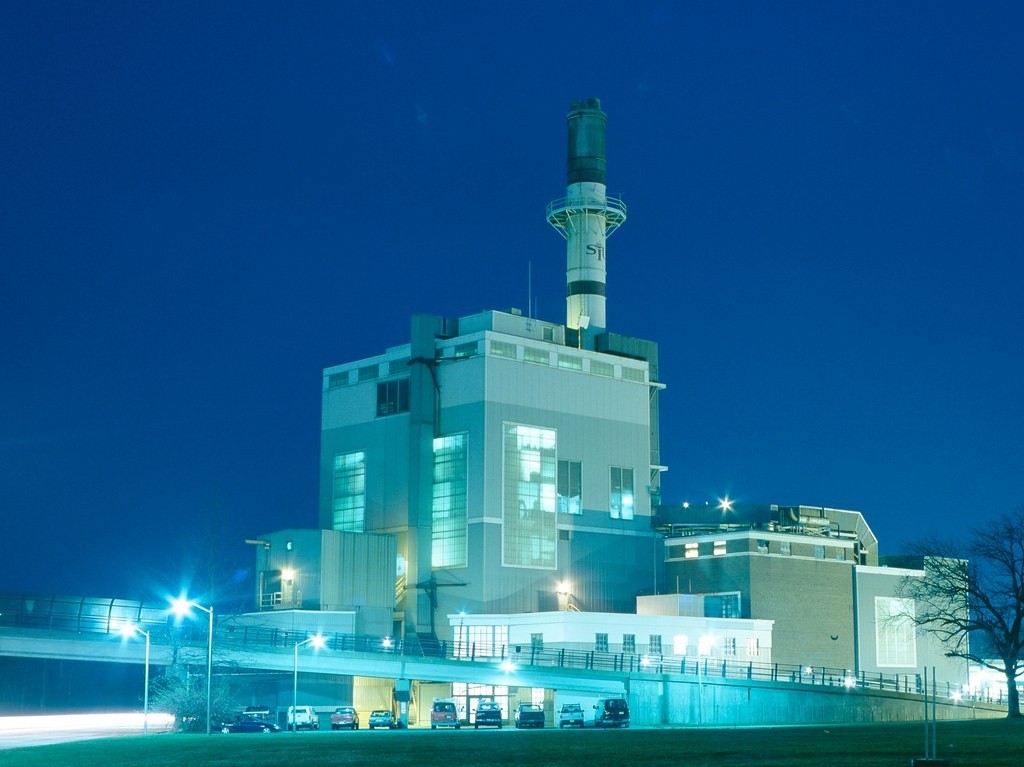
[118,621,149,734]
[292,631,328,733]
[169,597,213,734]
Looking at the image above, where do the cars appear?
[221,717,280,734]
[369,710,396,730]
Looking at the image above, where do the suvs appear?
[288,707,319,731]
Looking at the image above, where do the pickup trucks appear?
[331,707,359,731]
[558,702,585,728]
[241,706,273,721]
[514,703,546,729]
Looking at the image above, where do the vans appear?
[592,698,631,729]
[473,702,503,729]
[431,702,461,730]
